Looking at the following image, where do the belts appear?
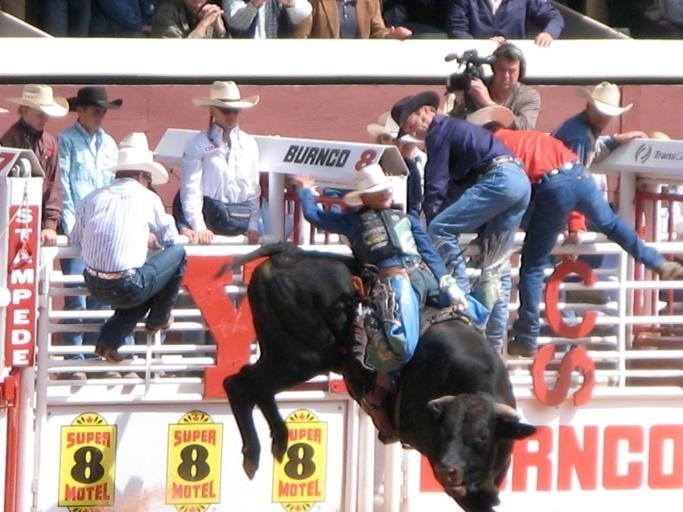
[84,264,140,280]
[534,158,584,188]
[484,154,523,172]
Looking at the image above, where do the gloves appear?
[437,275,469,312]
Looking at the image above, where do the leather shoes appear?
[359,391,396,441]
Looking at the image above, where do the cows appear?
[211,241,540,512]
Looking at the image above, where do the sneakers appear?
[144,314,174,334]
[506,342,539,357]
[94,340,125,362]
[68,371,179,381]
[652,262,683,281]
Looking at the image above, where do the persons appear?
[293,44,683,406]
[25,1,564,48]
[0,81,263,380]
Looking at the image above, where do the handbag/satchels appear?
[203,196,255,236]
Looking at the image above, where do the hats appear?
[106,131,168,187]
[192,80,262,111]
[67,86,127,111]
[365,90,441,143]
[342,162,404,207]
[574,80,636,119]
[463,104,515,131]
[5,84,70,119]
